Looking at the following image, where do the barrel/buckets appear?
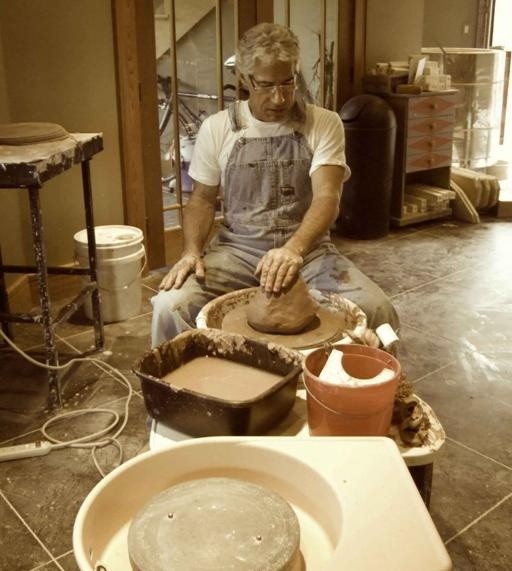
[73,224,146,322]
[303,345,402,436]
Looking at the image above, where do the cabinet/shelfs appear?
[365,90,460,228]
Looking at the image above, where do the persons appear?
[151,21,401,360]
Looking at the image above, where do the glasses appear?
[248,74,298,95]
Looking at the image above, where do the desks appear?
[0,132,105,409]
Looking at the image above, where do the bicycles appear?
[156,72,234,182]
